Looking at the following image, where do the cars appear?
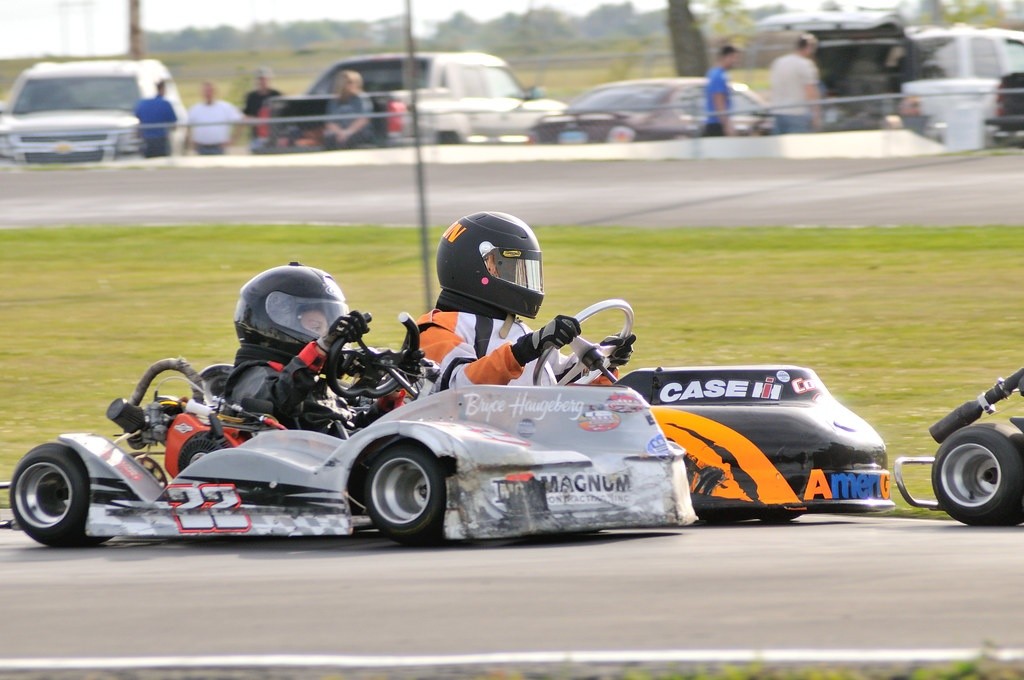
[758,8,1024,153]
[532,76,772,143]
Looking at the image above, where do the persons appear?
[243,68,285,154]
[186,79,258,155]
[323,70,376,149]
[136,80,177,157]
[704,46,740,137]
[224,261,426,436]
[768,34,823,133]
[401,211,636,393]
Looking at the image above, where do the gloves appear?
[329,310,370,343]
[599,332,636,367]
[517,315,582,366]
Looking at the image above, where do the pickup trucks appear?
[248,49,573,161]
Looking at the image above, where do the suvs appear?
[0,59,193,167]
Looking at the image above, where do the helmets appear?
[234,265,349,348]
[437,211,546,319]
